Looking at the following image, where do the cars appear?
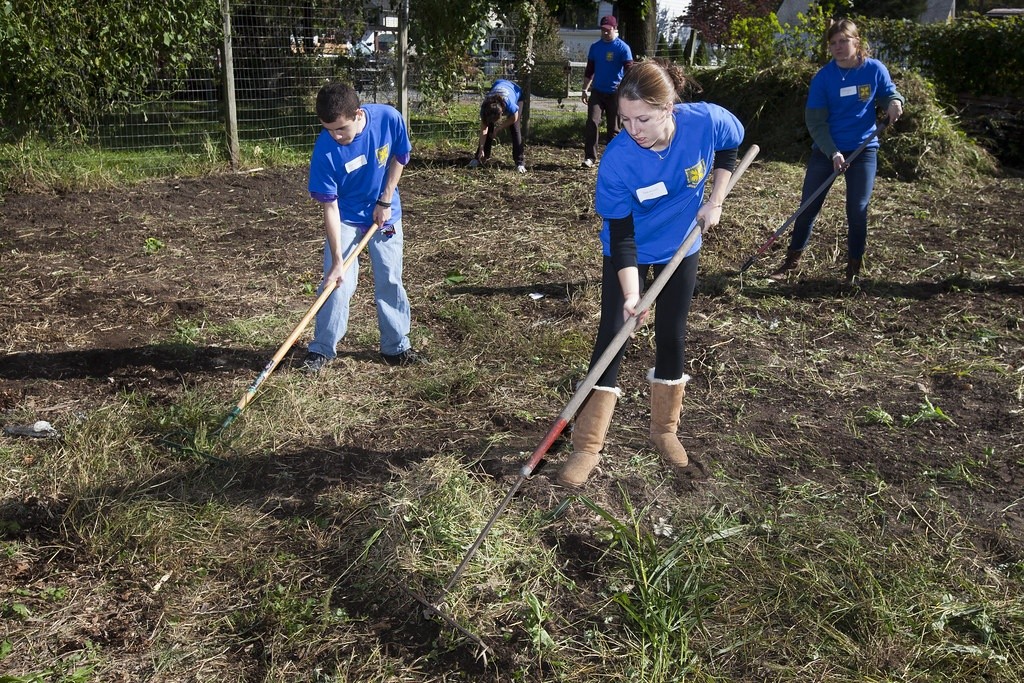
[321,41,396,89]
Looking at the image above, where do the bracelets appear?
[377,199,392,207]
[582,89,587,91]
[709,199,722,207]
[479,148,483,150]
[833,152,842,160]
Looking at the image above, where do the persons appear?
[581,16,634,168]
[298,84,426,373]
[558,63,744,489]
[766,20,904,281]
[469,80,527,173]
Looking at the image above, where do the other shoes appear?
[582,159,596,168]
[516,165,527,174]
[481,156,491,164]
[300,352,329,374]
[380,348,429,367]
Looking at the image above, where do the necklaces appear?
[652,117,676,159]
[837,66,850,81]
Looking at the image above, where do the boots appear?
[769,246,804,283]
[836,270,860,299]
[556,381,625,489]
[645,366,690,468]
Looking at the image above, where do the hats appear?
[599,15,618,30]
